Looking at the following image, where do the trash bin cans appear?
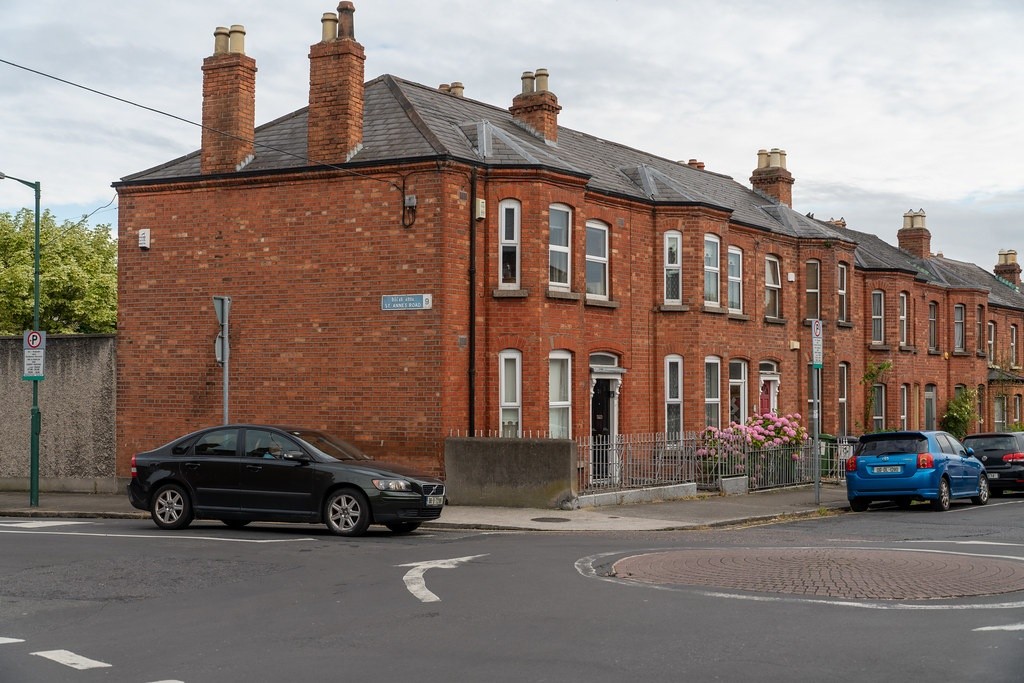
[835,436,860,478]
[811,434,837,477]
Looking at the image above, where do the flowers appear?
[697,412,808,489]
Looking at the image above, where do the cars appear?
[844,428,992,511]
[961,432,1024,497]
[126,421,447,536]
